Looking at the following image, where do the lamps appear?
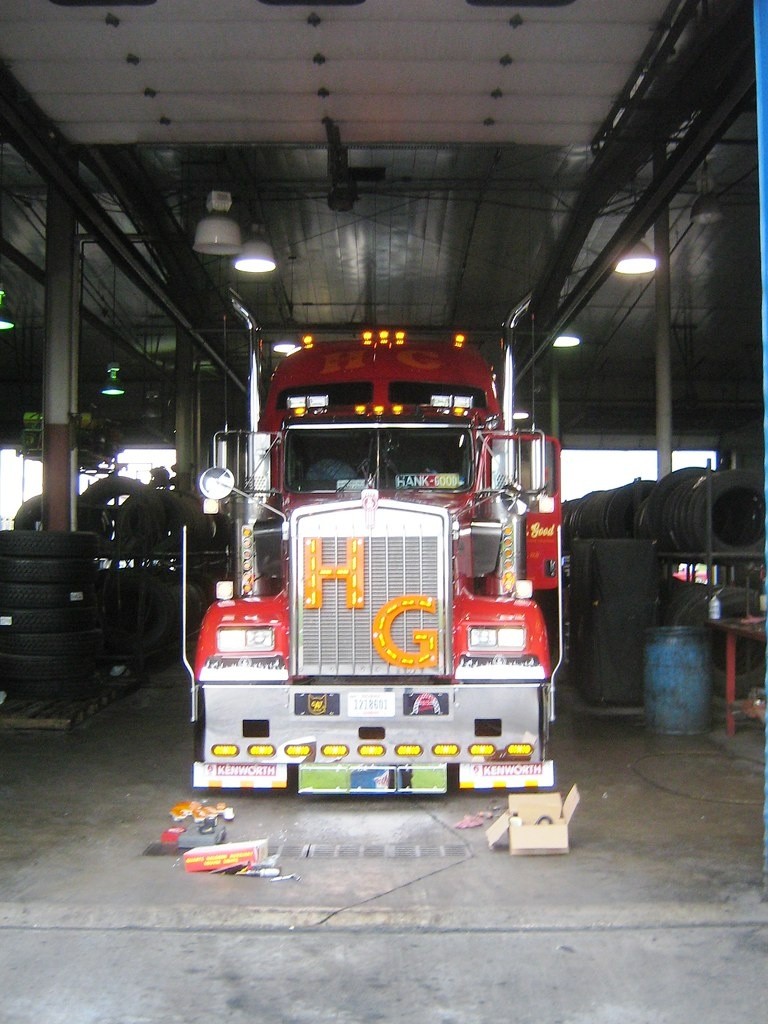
[192,188,276,273]
[551,158,729,349]
[512,403,530,420]
[0,284,15,330]
[100,264,126,396]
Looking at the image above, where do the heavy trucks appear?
[178,284,567,794]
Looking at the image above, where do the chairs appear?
[399,452,449,474]
[305,460,363,481]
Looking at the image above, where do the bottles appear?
[708,595,721,619]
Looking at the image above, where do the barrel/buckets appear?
[645,626,725,736]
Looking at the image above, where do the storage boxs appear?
[485,782,579,854]
